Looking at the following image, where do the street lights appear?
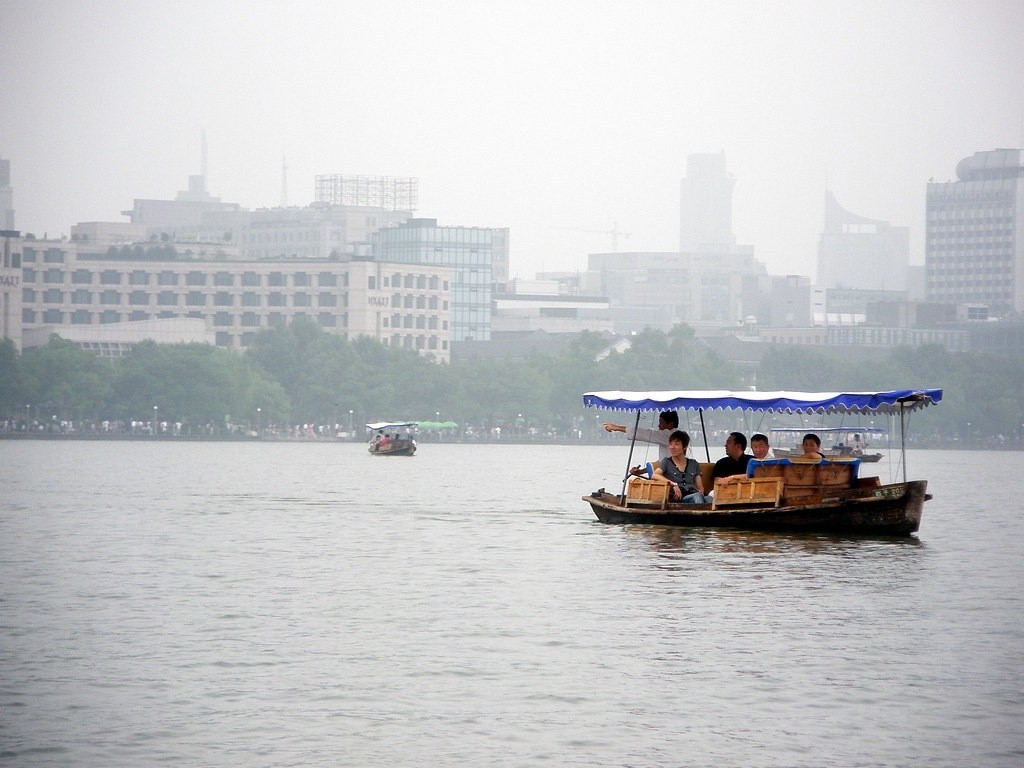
[257,408,262,437]
[349,410,353,435]
[26,404,31,432]
[436,411,442,422]
[154,406,162,435]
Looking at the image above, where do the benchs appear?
[746,456,863,498]
[647,462,716,496]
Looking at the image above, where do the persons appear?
[710,432,757,498]
[0,417,1024,455]
[603,409,693,474]
[652,430,713,507]
[801,433,826,459]
[748,433,773,459]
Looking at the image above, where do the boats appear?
[578,388,947,537]
[770,426,885,463]
[366,421,420,456]
